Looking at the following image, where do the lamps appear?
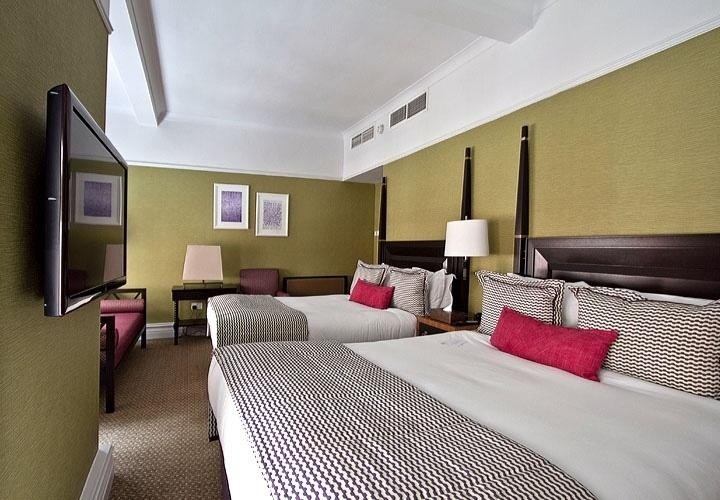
[443,215,490,325]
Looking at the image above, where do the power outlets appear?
[190,301,203,310]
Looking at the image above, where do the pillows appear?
[99,299,145,314]
[473,267,720,400]
[100,325,120,350]
[349,260,456,318]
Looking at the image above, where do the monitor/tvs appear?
[41,83,127,318]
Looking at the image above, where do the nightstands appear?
[416,315,480,336]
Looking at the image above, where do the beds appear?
[205,146,472,349]
[204,124,720,500]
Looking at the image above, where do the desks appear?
[171,286,238,346]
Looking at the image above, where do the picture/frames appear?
[255,192,290,238]
[212,182,250,230]
[74,171,123,226]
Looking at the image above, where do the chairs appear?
[239,268,291,297]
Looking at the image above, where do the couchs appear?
[99,288,146,413]
